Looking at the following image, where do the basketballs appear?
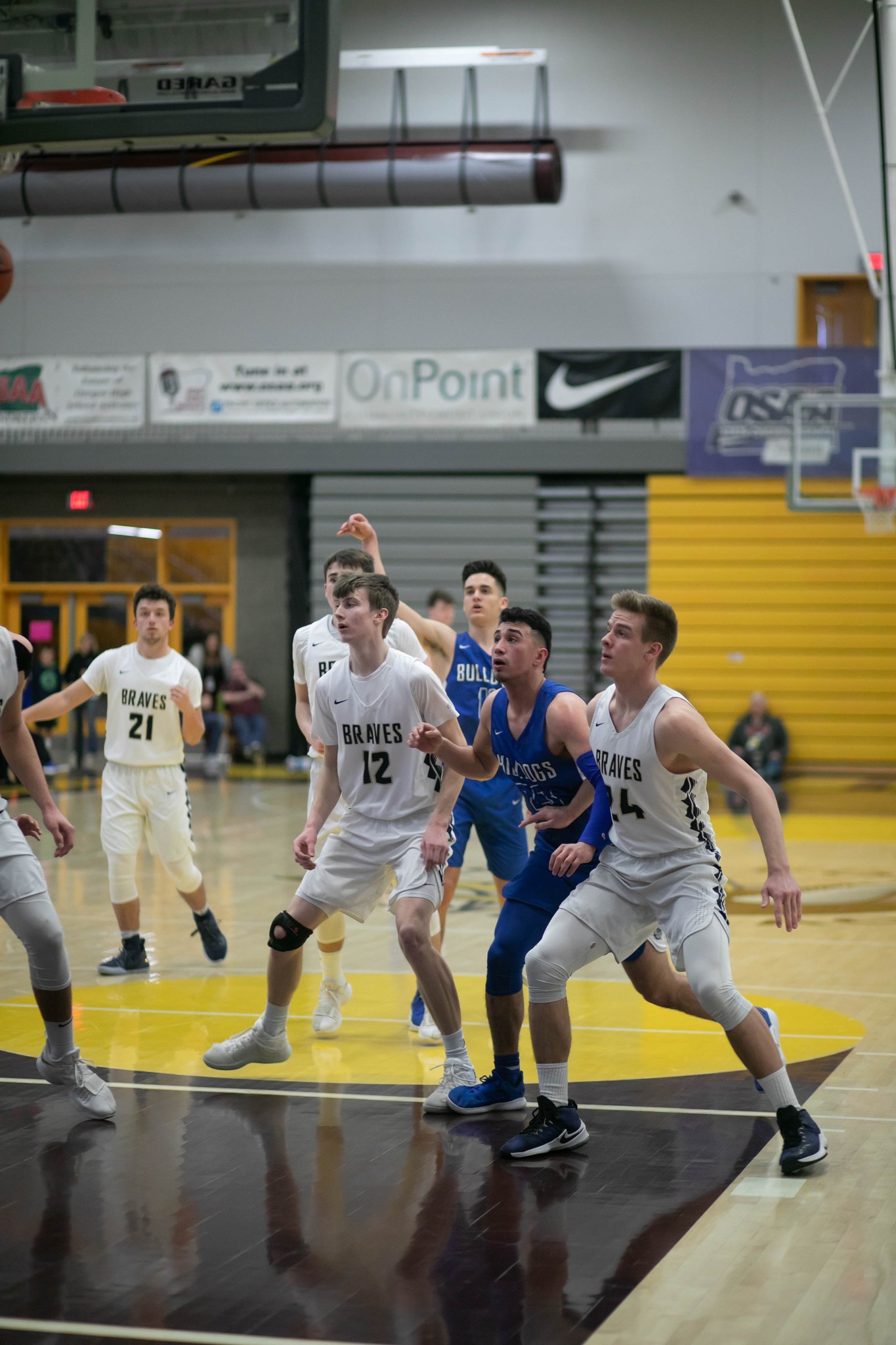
[0,240,14,302]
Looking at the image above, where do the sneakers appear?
[776,1106,829,1173]
[497,1153,588,1201]
[499,1095,590,1159]
[36,1030,116,1119]
[756,1006,787,1093]
[423,1112,476,1154]
[190,909,227,963]
[423,1057,480,1113]
[445,1111,526,1146]
[419,1012,442,1039]
[97,935,150,975]
[446,1070,527,1115]
[409,988,425,1031]
[312,982,352,1032]
[35,1120,116,1169]
[203,1014,293,1071]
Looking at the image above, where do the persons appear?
[0,550,826,1175]
[337,513,537,1031]
